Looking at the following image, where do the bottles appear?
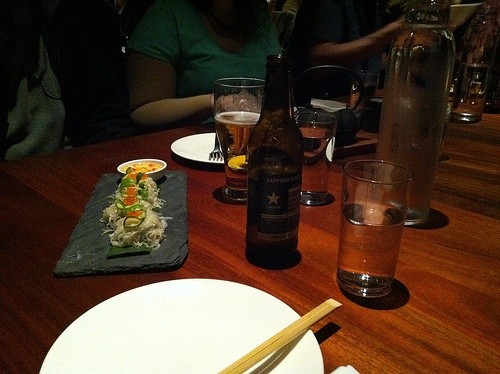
[368,0,457,225]
[449,0,500,124]
[245,52,305,268]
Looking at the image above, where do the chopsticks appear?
[222,297,342,374]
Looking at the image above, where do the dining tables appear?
[0,102,500,374]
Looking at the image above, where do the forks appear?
[209,134,223,162]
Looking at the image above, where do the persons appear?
[124,0,283,130]
[292,0,404,106]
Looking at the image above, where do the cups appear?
[345,70,378,129]
[213,76,265,204]
[439,78,457,161]
[336,158,411,298]
[295,111,336,205]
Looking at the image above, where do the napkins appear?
[310,98,346,112]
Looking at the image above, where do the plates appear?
[116,159,167,182]
[54,170,188,275]
[39,278,326,374]
[310,98,345,113]
[171,132,224,164]
